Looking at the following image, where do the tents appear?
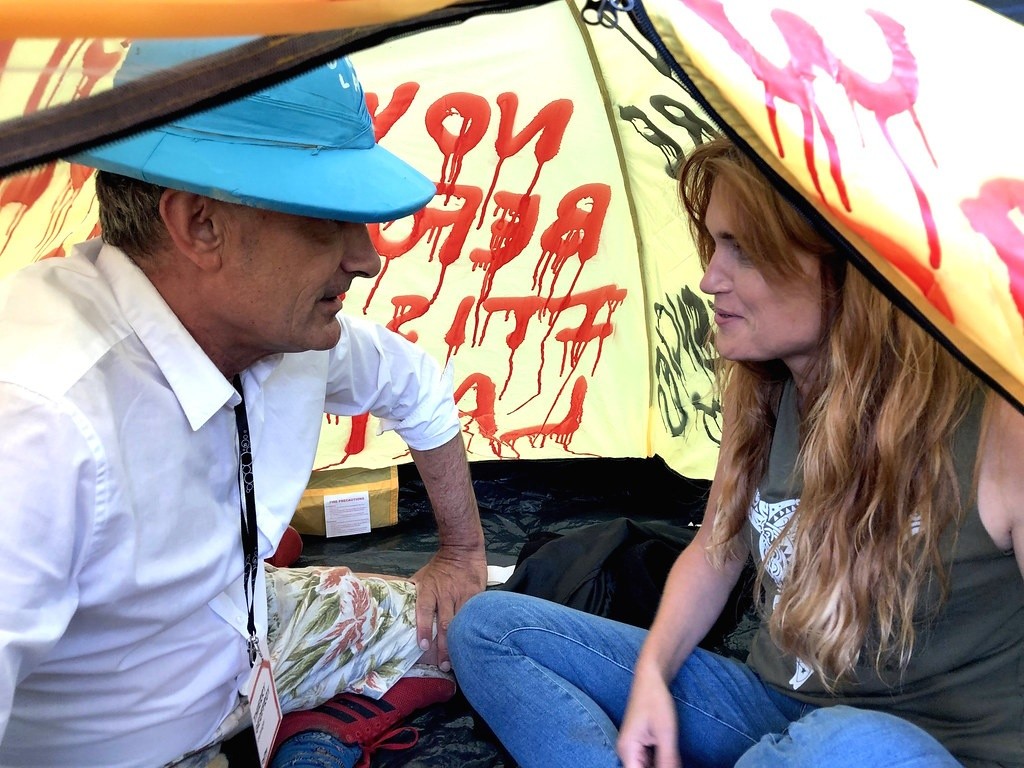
[0,0,1024,425]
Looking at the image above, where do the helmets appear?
[60,40,437,222]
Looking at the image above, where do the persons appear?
[443,133,1024,768]
[0,38,489,767]
